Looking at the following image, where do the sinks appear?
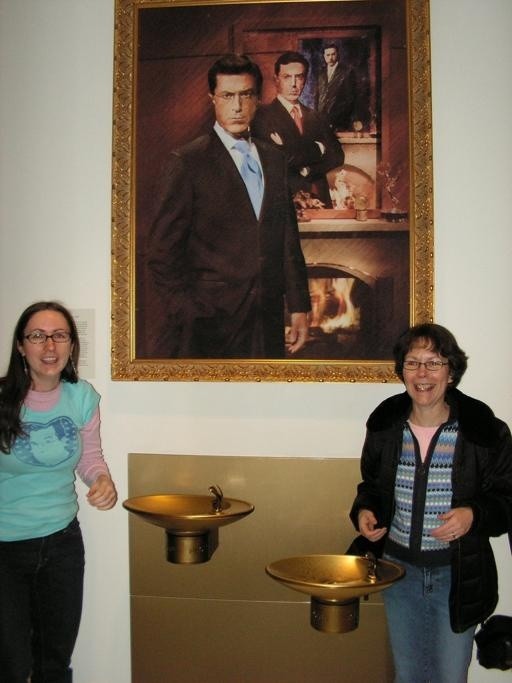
[121,493,255,532]
[263,553,406,604]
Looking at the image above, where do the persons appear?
[1,302,117,683]
[341,324,511,682]
[250,51,345,210]
[146,51,313,358]
[315,40,369,132]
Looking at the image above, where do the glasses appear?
[24,332,71,344]
[403,360,449,370]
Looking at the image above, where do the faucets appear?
[208,484,224,513]
[365,552,381,581]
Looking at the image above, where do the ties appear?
[234,140,263,217]
[293,107,305,137]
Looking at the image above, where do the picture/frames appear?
[111,0,438,385]
